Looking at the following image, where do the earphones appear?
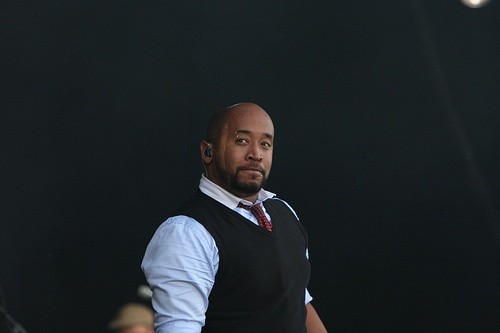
[205,148,211,157]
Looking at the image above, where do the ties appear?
[237,202,273,233]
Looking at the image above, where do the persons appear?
[139,102,328,333]
